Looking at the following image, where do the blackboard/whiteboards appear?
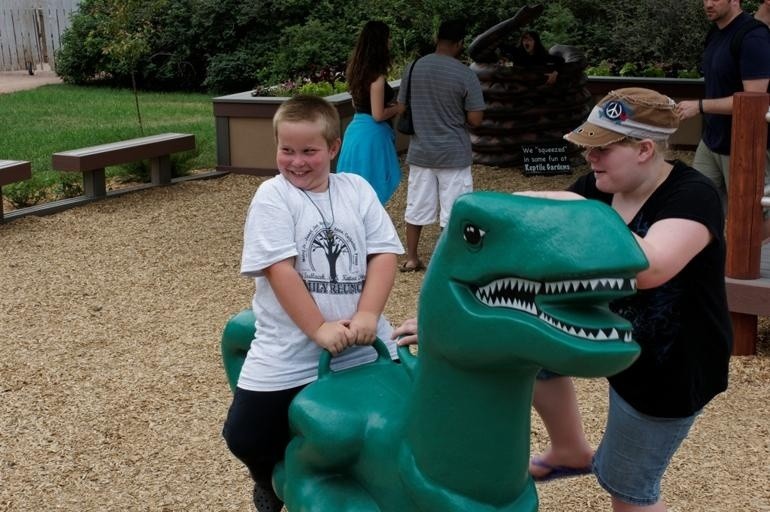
[521,144,571,177]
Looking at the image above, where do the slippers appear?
[528,450,598,483]
[399,258,426,273]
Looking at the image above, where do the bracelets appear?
[698,97,704,115]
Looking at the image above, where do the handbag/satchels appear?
[394,89,414,136]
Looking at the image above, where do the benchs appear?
[52,133,195,198]
[0,160,31,218]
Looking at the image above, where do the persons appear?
[515,31,586,85]
[525,87,733,511]
[395,13,487,273]
[750,1,770,30]
[665,1,770,192]
[337,20,407,206]
[219,93,407,511]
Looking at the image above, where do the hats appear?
[560,84,683,151]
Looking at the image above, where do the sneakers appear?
[252,486,286,512]
